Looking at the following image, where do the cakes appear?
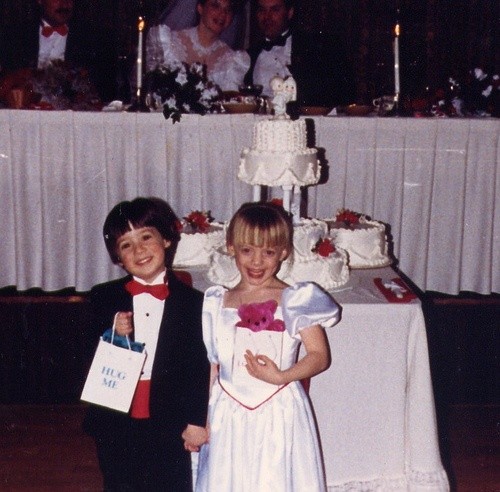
[209,199,349,290]
[172,211,228,266]
[237,76,322,186]
[321,209,389,265]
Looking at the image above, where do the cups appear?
[146,94,161,111]
[13,90,26,109]
[373,97,394,115]
[240,84,264,98]
[451,96,464,115]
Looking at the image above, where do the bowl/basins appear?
[223,103,259,113]
[343,106,370,116]
[298,107,330,115]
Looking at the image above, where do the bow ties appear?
[124,275,171,300]
[262,29,291,51]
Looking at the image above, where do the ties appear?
[38,17,69,37]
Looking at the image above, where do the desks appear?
[0,110,500,294]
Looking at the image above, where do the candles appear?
[136,20,145,88]
[393,23,400,102]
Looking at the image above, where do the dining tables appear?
[174,267,450,492]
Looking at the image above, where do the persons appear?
[145,0,251,92]
[244,0,321,105]
[202,202,340,492]
[77,198,211,492]
[16,0,85,72]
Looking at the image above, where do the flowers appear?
[335,209,360,227]
[180,208,214,234]
[312,235,337,256]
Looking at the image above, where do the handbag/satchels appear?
[81,313,147,414]
